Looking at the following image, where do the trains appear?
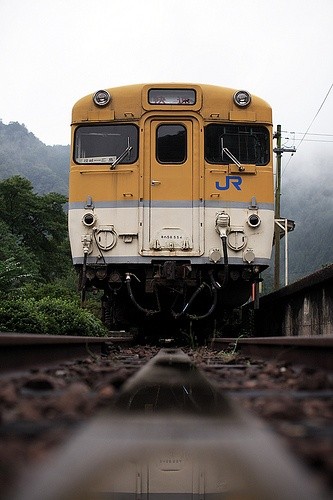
[65,82,278,351]
